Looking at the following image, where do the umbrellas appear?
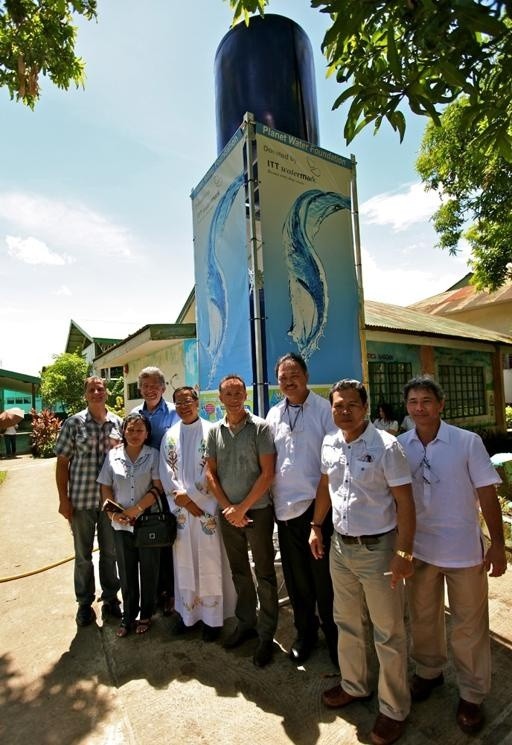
[0,407,25,429]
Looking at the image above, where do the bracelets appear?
[396,549,416,565]
[136,503,143,513]
[310,522,322,528]
[111,511,118,523]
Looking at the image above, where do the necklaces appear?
[286,407,302,435]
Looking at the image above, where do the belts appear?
[275,518,304,527]
[335,527,401,546]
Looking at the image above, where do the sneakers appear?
[199,620,222,643]
[101,598,122,623]
[75,603,92,627]
[171,616,201,636]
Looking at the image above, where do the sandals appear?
[135,617,153,635]
[115,619,135,638]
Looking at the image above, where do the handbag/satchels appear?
[130,486,178,550]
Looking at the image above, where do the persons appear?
[399,414,416,435]
[206,376,278,668]
[397,375,508,736]
[265,352,342,673]
[96,414,171,637]
[372,402,399,436]
[4,424,19,459]
[159,387,238,642]
[129,366,182,615]
[308,377,416,745]
[53,375,126,627]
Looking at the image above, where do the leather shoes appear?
[252,637,274,668]
[368,711,408,745]
[322,685,374,709]
[288,630,319,664]
[456,697,485,734]
[322,636,340,669]
[220,625,260,649]
[410,669,445,704]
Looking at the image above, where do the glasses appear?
[174,398,196,407]
